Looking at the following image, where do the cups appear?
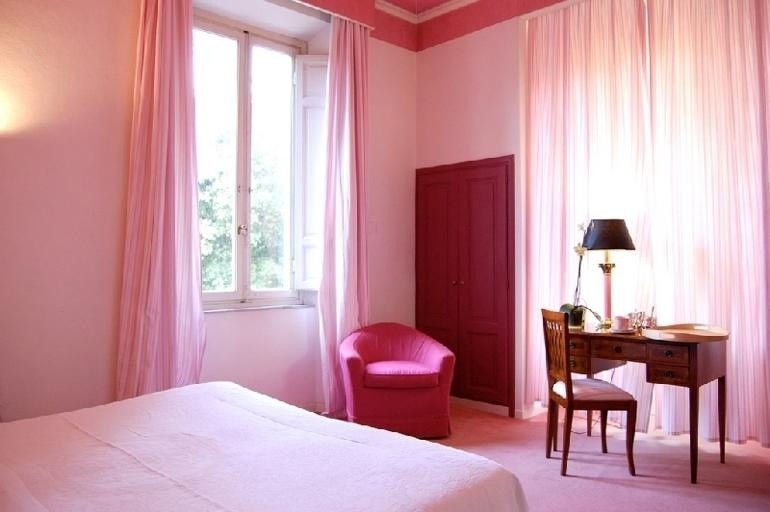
[615,316,628,331]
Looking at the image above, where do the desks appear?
[551,319,730,486]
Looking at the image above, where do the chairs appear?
[540,305,638,480]
[337,322,456,442]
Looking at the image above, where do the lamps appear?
[581,218,637,330]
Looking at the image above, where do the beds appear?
[2,380,533,511]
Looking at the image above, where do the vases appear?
[570,310,584,326]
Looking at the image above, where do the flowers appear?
[558,222,604,322]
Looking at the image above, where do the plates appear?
[609,327,637,335]
